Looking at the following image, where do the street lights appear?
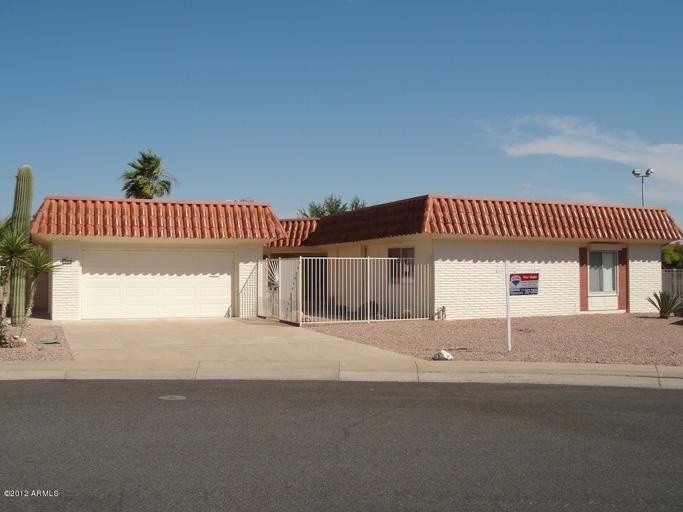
[631,163,655,209]
